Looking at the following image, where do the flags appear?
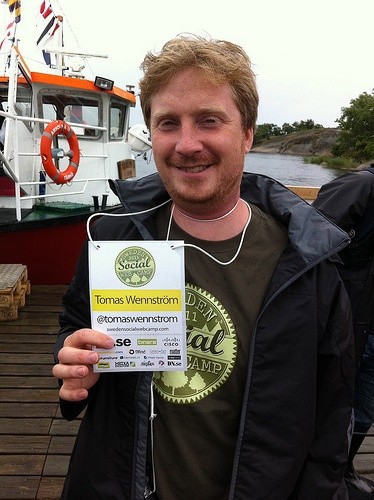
[1,0,21,23]
[0,19,15,48]
[36,16,60,49]
[40,0,52,18]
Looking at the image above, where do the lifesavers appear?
[40,120,80,184]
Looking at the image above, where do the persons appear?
[312,167,374,500]
[51,31,357,500]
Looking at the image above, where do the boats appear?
[0,0,159,286]
